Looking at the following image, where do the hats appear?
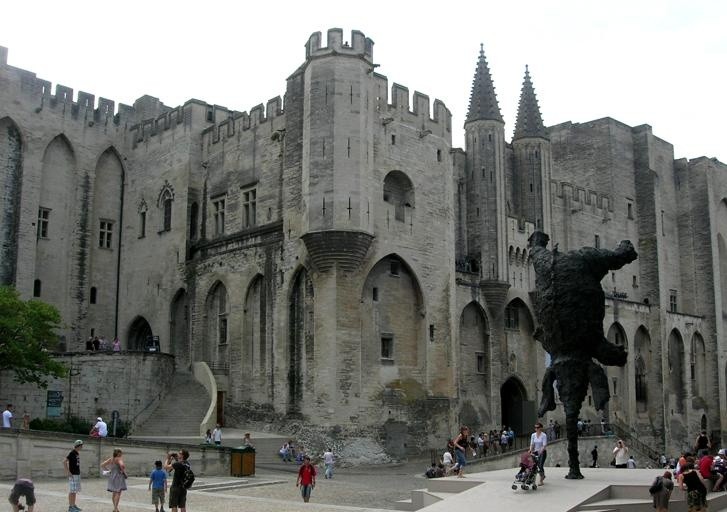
[74,440,83,446]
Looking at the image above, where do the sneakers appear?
[458,474,466,479]
[537,481,544,486]
[68,506,80,512]
[75,505,83,511]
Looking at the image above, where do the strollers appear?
[511,449,543,490]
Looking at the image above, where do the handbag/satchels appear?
[649,482,662,493]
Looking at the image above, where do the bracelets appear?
[313,482,315,485]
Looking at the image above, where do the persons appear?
[204,429,213,445]
[277,438,307,463]
[89,424,99,439]
[163,447,192,512]
[678,463,706,511]
[242,431,256,449]
[213,424,222,446]
[7,478,37,512]
[88,417,109,439]
[63,439,84,512]
[296,457,316,502]
[85,335,121,353]
[2,404,14,429]
[99,448,129,512]
[649,472,673,511]
[323,448,336,480]
[686,462,708,511]
[147,459,169,512]
[21,410,29,431]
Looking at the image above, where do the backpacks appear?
[179,461,195,490]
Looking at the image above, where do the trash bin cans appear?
[230,445,256,477]
